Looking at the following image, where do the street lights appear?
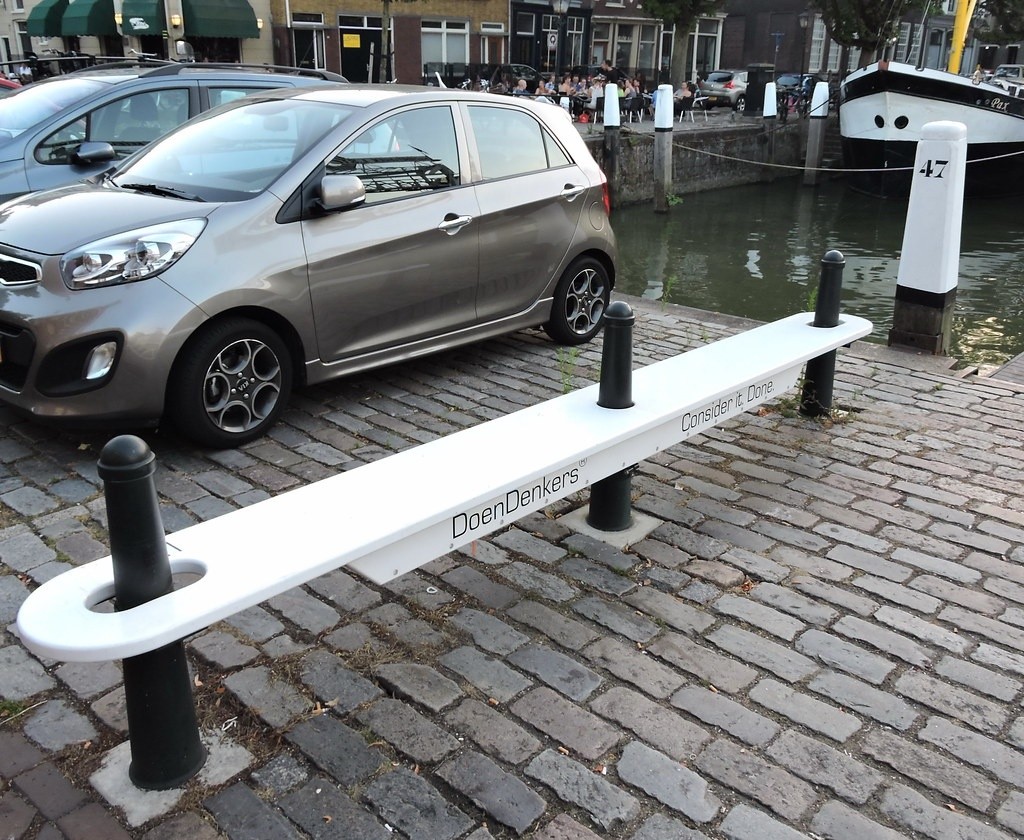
[551,0,571,91]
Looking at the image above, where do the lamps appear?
[115,14,123,27]
[257,19,263,31]
[172,14,181,28]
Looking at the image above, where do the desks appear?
[495,93,655,99]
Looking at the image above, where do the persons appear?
[674,81,697,103]
[20,63,32,84]
[203,57,208,62]
[534,60,642,122]
[974,64,986,79]
[513,79,530,99]
[650,82,664,121]
[1,71,7,76]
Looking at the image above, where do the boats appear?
[839,0,1024,199]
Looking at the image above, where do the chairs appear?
[434,71,449,89]
[347,128,375,158]
[552,91,709,124]
[119,94,161,141]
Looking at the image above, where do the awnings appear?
[27,0,70,38]
[122,0,167,36]
[62,0,117,36]
[182,0,260,38]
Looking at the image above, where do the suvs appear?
[0,61,399,206]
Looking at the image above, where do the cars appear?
[697,70,786,113]
[777,74,824,98]
[464,63,550,93]
[570,66,632,82]
[0,78,87,138]
[0,83,618,454]
